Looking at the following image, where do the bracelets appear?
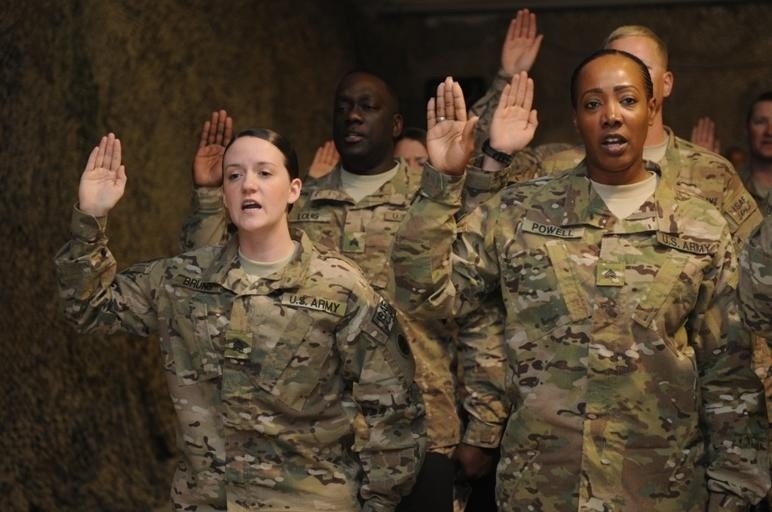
[481,139,515,166]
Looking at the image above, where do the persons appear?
[182,70,507,512]
[392,48,772,512]
[725,146,745,171]
[466,8,772,413]
[688,90,772,228]
[51,129,430,511]
[300,133,428,194]
[454,71,554,225]
[736,210,772,353]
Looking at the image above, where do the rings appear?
[435,117,447,123]
